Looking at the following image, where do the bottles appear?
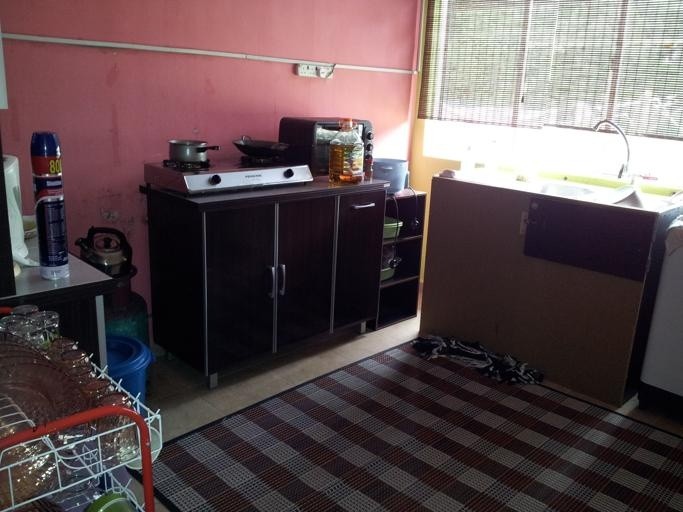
[30,130,71,282]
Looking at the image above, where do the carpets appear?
[123,328,683,512]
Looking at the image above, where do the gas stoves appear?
[143,157,315,194]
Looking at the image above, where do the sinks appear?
[541,180,683,212]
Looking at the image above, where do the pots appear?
[167,138,220,163]
[231,135,291,159]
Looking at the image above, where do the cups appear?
[609,184,645,210]
[88,492,137,512]
[122,426,162,471]
[93,392,139,464]
[0,304,60,352]
[83,378,113,424]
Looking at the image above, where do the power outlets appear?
[293,61,337,79]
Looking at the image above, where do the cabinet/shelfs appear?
[378,189,429,330]
[1,303,166,512]
[134,181,389,390]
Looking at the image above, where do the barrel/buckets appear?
[107,332,156,414]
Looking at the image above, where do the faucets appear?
[593,119,630,174]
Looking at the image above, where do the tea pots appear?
[74,226,133,279]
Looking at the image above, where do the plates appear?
[0,325,91,509]
[22,216,37,239]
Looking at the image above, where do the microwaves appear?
[277,115,374,176]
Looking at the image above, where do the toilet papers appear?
[3,153,41,268]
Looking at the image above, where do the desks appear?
[0,231,123,398]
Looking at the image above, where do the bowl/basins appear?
[381,265,396,280]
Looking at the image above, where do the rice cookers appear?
[370,157,409,195]
